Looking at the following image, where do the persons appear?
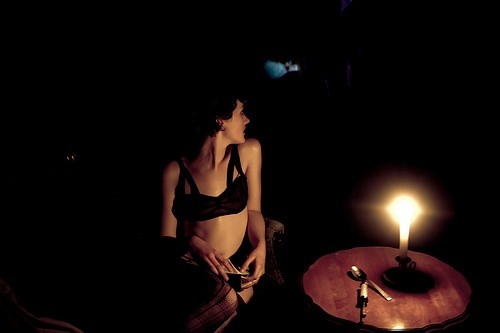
[161,80,267,311]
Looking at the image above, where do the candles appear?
[388,192,423,261]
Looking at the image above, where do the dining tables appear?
[302,246,473,333]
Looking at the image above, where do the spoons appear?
[351,265,392,301]
